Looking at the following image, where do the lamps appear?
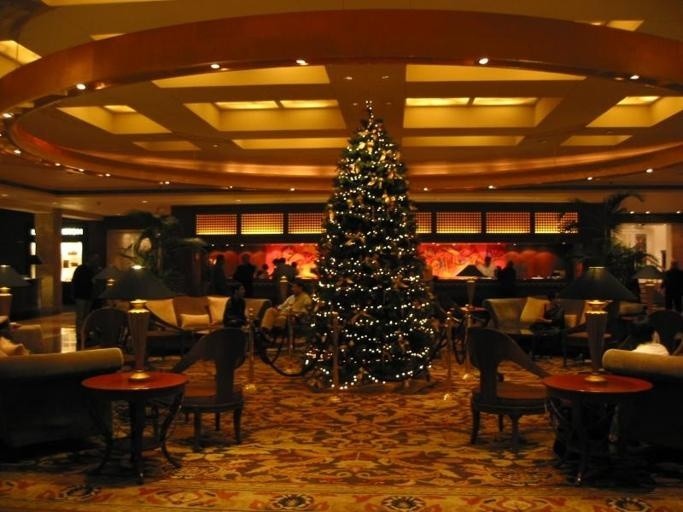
[461,266,488,308]
[99,265,176,380]
[632,265,663,314]
[559,267,635,383]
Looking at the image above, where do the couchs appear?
[120,297,271,346]
[0,317,125,465]
[483,297,647,359]
[600,350,683,483]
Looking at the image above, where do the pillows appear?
[180,313,211,327]
[519,297,551,325]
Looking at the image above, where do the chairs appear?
[80,308,135,369]
[160,330,251,451]
[465,325,548,450]
[254,306,310,358]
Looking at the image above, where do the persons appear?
[496,262,516,298]
[209,254,313,338]
[661,262,683,314]
[530,289,565,357]
[70,251,105,344]
[477,256,496,277]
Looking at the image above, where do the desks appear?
[80,372,184,490]
[459,307,487,371]
[209,319,251,351]
[537,372,651,486]
